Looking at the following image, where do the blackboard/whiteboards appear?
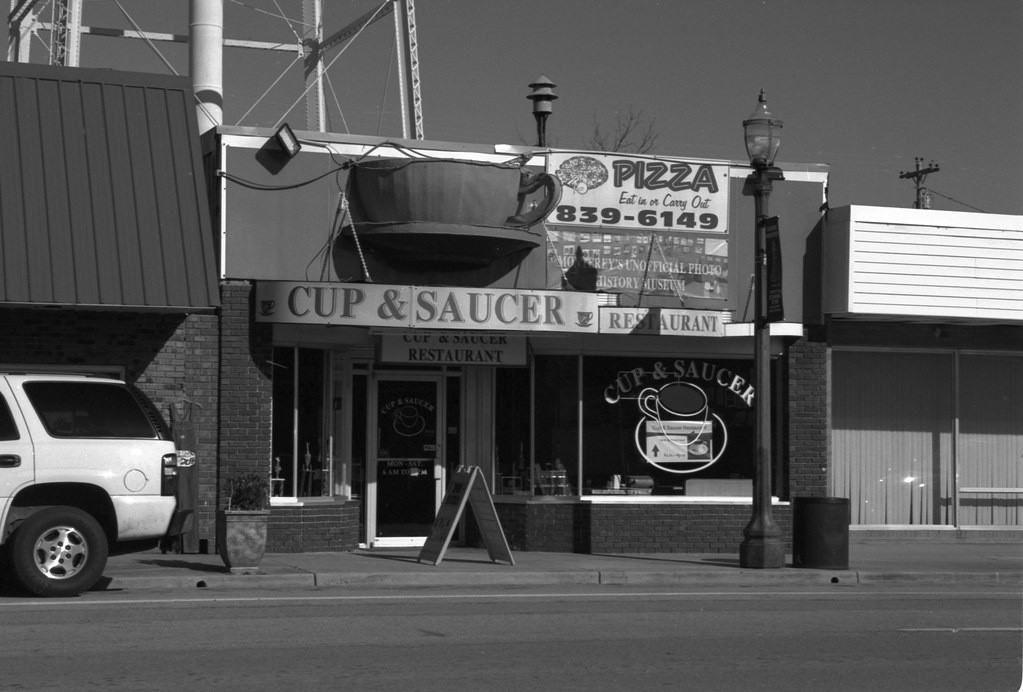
[418,466,477,556]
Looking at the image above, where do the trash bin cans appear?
[791,495,852,570]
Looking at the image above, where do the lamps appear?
[275,123,303,158]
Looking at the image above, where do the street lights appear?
[739,86,788,571]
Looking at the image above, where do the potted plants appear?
[216,471,271,577]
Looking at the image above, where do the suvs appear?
[0,368,179,598]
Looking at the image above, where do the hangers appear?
[164,387,204,410]
